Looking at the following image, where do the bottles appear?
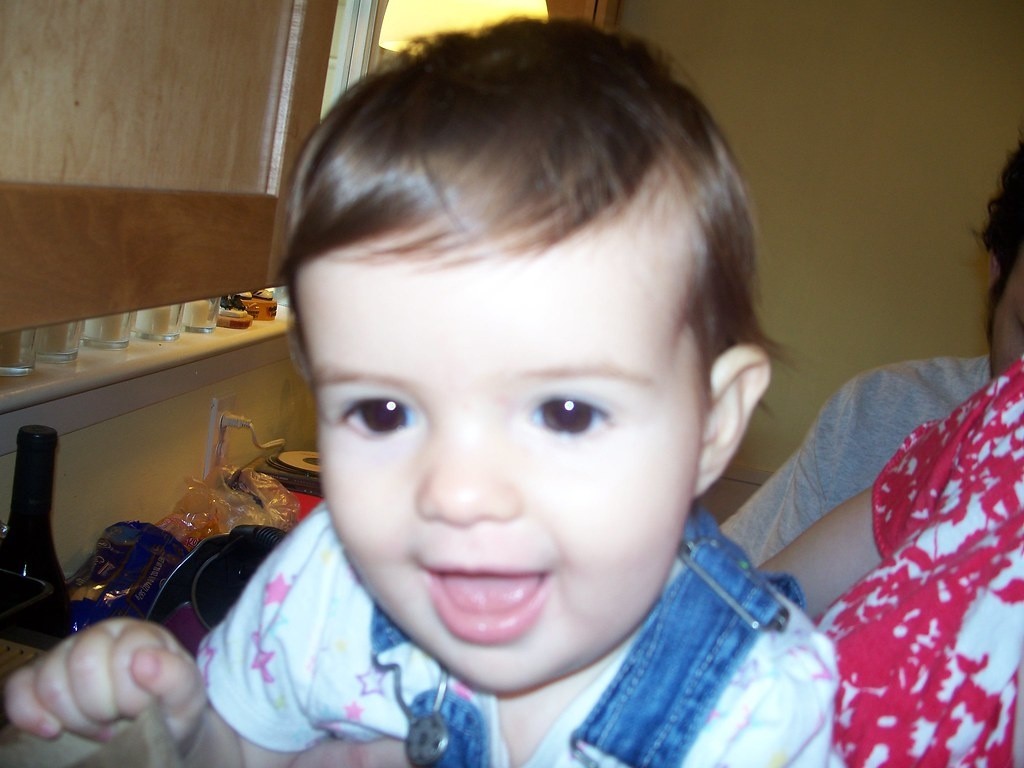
[0,425,74,651]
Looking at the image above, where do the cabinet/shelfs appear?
[0,0,339,336]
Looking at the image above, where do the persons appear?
[289,136,1023,768]
[0,20,846,768]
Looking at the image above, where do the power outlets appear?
[204,396,230,479]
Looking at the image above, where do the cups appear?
[135,303,184,341]
[81,311,133,349]
[183,296,221,334]
[0,328,41,376]
[36,320,85,363]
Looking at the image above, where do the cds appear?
[277,450,321,472]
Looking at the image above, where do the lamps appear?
[378,1,550,59]
